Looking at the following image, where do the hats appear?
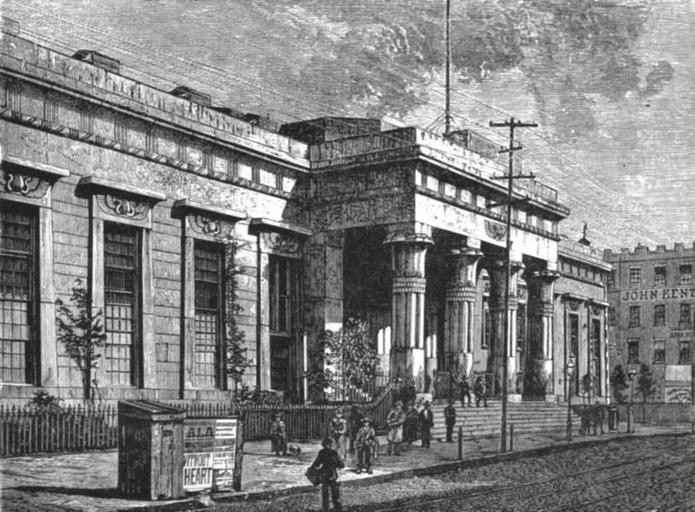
[461,375,468,379]
[423,400,430,406]
[448,399,455,404]
[360,417,373,423]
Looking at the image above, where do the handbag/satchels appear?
[271,435,281,446]
[306,468,324,486]
[355,442,364,449]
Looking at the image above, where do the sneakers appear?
[357,467,373,474]
